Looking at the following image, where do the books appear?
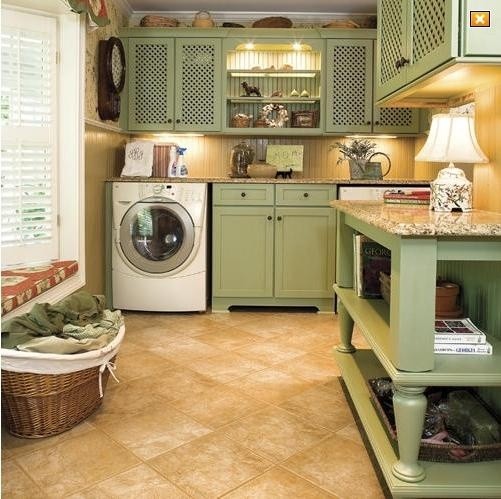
[434,317,492,356]
[384,189,431,205]
[353,233,390,298]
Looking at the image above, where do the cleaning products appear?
[168,146,177,177]
[176,148,189,177]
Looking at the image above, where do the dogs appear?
[240,81,262,97]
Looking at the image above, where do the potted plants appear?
[435,275,464,319]
[329,139,378,179]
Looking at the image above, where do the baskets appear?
[140,15,177,26]
[0,311,125,439]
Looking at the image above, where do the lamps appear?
[414,112,489,212]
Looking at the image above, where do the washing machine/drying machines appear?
[111,181,207,313]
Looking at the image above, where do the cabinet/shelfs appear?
[421,108,443,133]
[212,183,337,313]
[222,38,323,133]
[325,38,420,134]
[375,0,501,108]
[128,37,223,132]
[328,199,501,499]
